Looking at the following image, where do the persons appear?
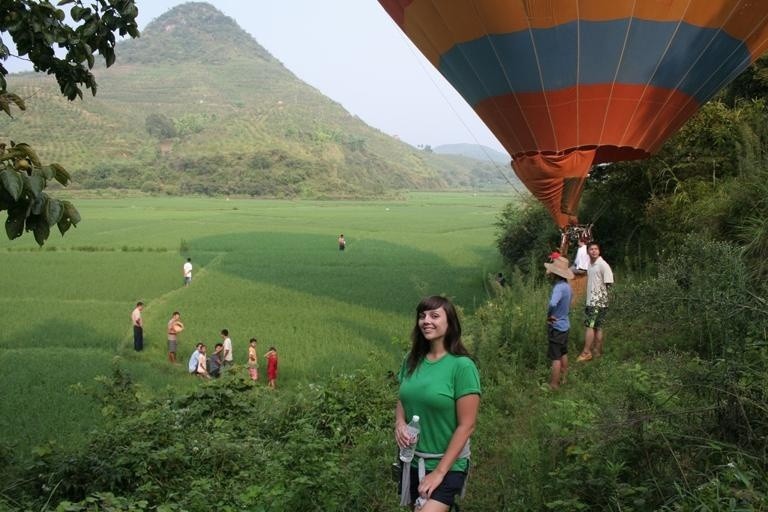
[187,328,235,383]
[337,233,347,251]
[575,240,616,363]
[130,300,145,352]
[263,346,279,389]
[182,257,193,288]
[247,337,259,381]
[166,311,185,366]
[393,295,482,512]
[542,255,575,394]
[568,238,592,276]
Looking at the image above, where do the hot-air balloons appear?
[371,0,767,310]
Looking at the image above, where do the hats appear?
[544,257,575,279]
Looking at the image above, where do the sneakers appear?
[576,354,592,362]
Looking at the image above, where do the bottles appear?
[398,414,421,463]
